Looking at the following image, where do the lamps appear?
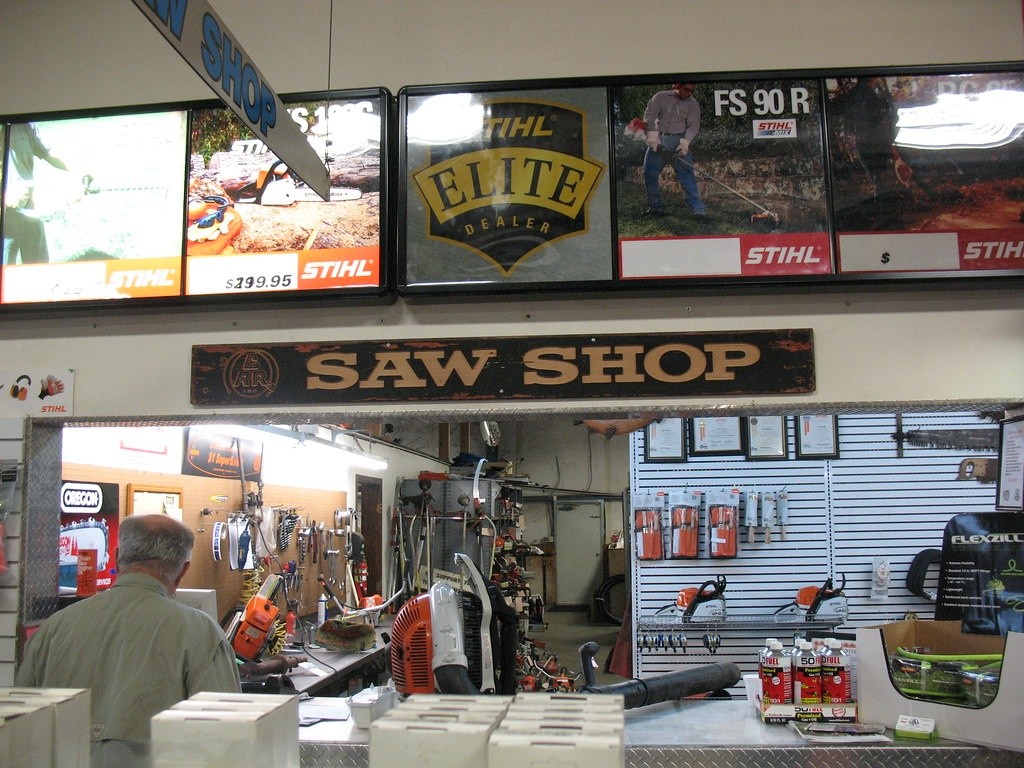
[191,424,388,471]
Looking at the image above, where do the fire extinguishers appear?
[352,555,370,600]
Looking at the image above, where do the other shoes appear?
[693,202,706,215]
[642,205,665,217]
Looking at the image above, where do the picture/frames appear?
[127,484,185,523]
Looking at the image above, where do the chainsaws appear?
[217,574,284,663]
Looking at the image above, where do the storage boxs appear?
[759,620,1024,753]
[0,685,626,768]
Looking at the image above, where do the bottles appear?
[757,637,851,705]
[317,593,328,627]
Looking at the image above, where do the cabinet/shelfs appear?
[401,479,522,578]
[608,549,626,621]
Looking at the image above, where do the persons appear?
[830,75,903,230]
[14,513,244,768]
[642,84,705,221]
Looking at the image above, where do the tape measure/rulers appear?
[637,633,722,653]
